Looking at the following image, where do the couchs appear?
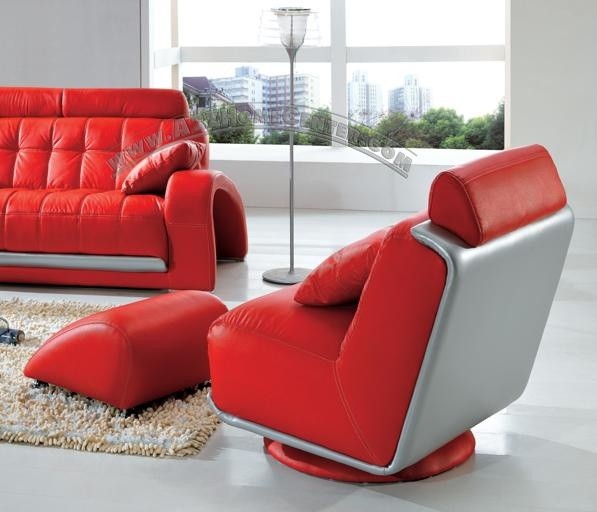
[0,86,248,296]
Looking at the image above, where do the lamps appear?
[260,9,311,289]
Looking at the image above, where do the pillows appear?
[295,222,393,309]
[120,141,205,196]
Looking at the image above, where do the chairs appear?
[208,140,586,487]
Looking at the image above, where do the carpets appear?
[2,297,219,458]
